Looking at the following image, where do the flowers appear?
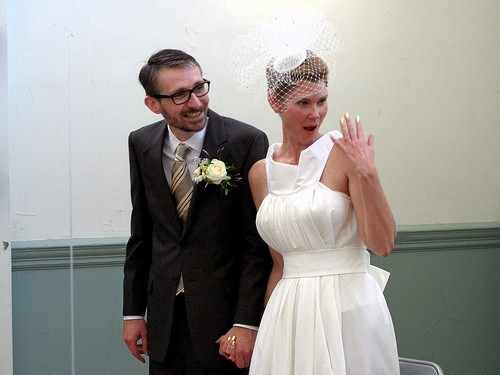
[191,147,243,196]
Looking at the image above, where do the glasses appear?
[150,78,211,105]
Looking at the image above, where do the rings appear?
[343,136,351,142]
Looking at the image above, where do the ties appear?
[171,144,195,296]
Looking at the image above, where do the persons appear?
[122,48,273,375]
[215,48,402,375]
